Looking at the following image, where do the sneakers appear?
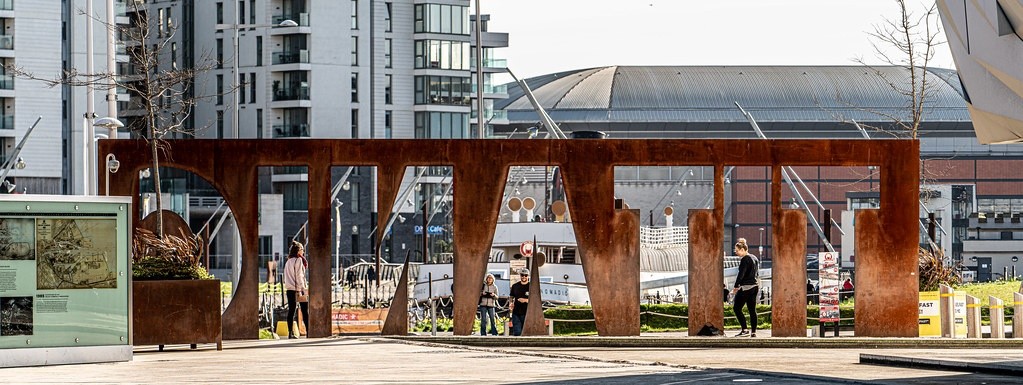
[735,330,757,338]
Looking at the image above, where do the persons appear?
[843,278,854,298]
[723,283,730,305]
[656,291,661,304]
[509,268,531,336]
[675,289,682,297]
[480,273,499,337]
[346,267,355,287]
[732,237,761,338]
[806,279,816,305]
[366,265,375,285]
[284,239,310,341]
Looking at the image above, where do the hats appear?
[520,269,529,274]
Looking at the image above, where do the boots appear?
[289,331,298,338]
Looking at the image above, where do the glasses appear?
[487,277,493,279]
[521,274,528,277]
[736,249,740,252]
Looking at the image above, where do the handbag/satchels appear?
[297,295,308,302]
